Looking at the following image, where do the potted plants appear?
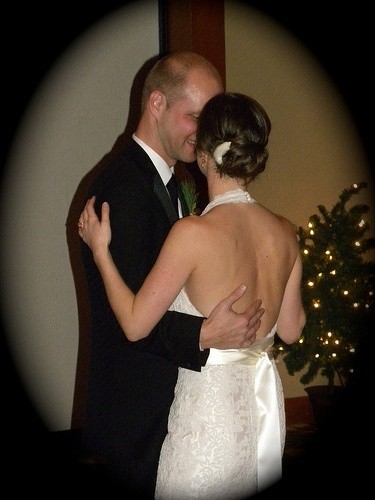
[273,183,375,431]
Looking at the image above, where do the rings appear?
[78,223,83,227]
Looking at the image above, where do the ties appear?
[166,173,181,221]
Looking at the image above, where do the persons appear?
[68,51,265,500]
[79,92,307,500]
